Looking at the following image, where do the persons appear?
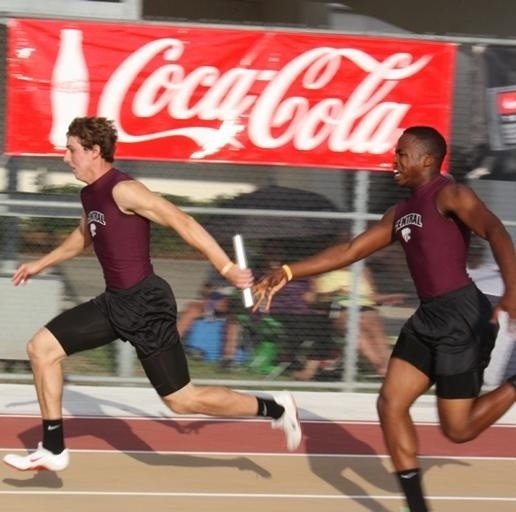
[246,126,516,512]
[3,117,303,470]
[250,229,406,379]
[175,279,245,367]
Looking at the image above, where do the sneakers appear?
[271,390,302,452]
[2,440,69,472]
